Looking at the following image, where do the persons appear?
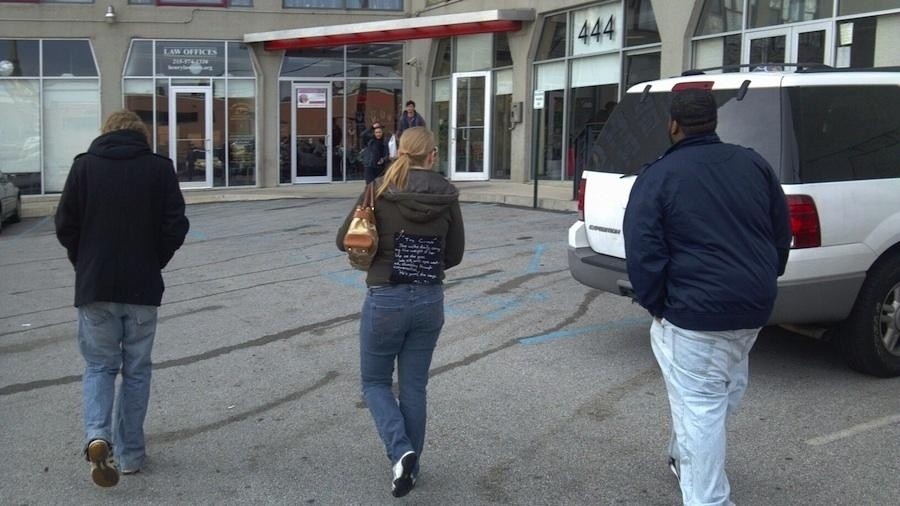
[362,125,391,186]
[54,109,191,488]
[395,100,426,151]
[333,125,466,496]
[619,87,792,506]
[361,115,397,169]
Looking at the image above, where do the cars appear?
[187,146,224,179]
[0,169,22,234]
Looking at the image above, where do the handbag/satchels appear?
[342,204,379,271]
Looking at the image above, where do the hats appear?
[669,90,717,126]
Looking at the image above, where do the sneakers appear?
[391,449,417,497]
[88,438,120,488]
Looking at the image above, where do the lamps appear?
[105,7,117,25]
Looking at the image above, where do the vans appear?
[566,62,900,380]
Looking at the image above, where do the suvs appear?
[220,139,256,175]
